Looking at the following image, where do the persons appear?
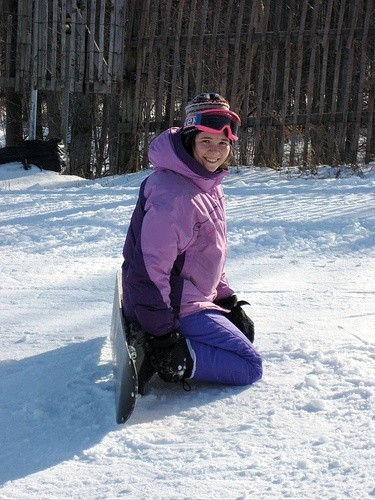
[121,91,262,394]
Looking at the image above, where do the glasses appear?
[183,109,241,142]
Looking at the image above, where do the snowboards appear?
[110,270,142,424]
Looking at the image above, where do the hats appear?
[181,93,230,136]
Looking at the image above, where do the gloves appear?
[158,326,196,391]
[213,295,254,344]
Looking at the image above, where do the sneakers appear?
[134,345,156,396]
